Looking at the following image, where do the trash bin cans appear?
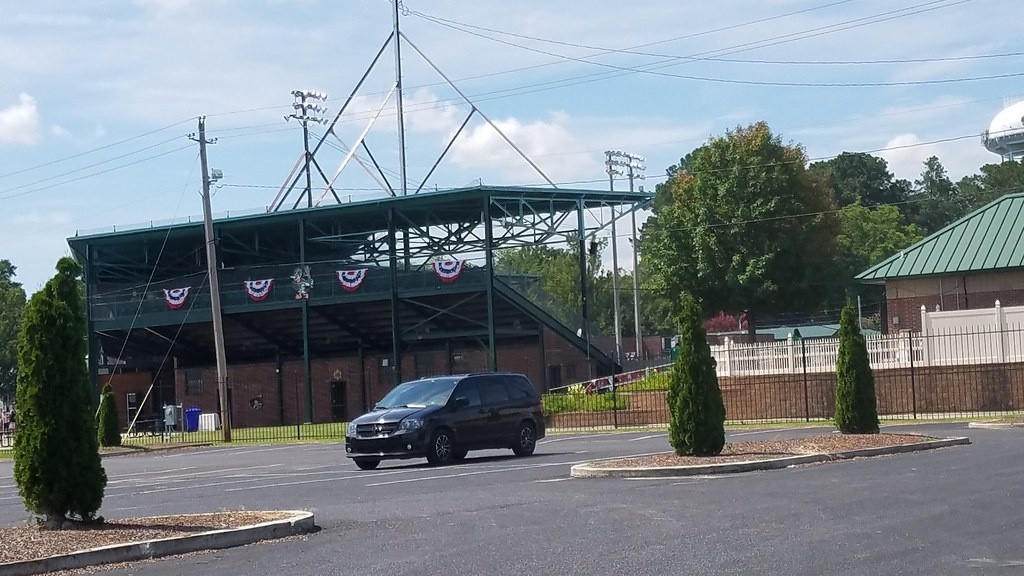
[185,407,202,433]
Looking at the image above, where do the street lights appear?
[605,149,628,362]
[282,89,328,209]
[622,149,647,364]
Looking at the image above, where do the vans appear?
[345,372,545,466]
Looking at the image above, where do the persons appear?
[671,343,679,361]
[792,329,802,341]
[0,406,17,432]
